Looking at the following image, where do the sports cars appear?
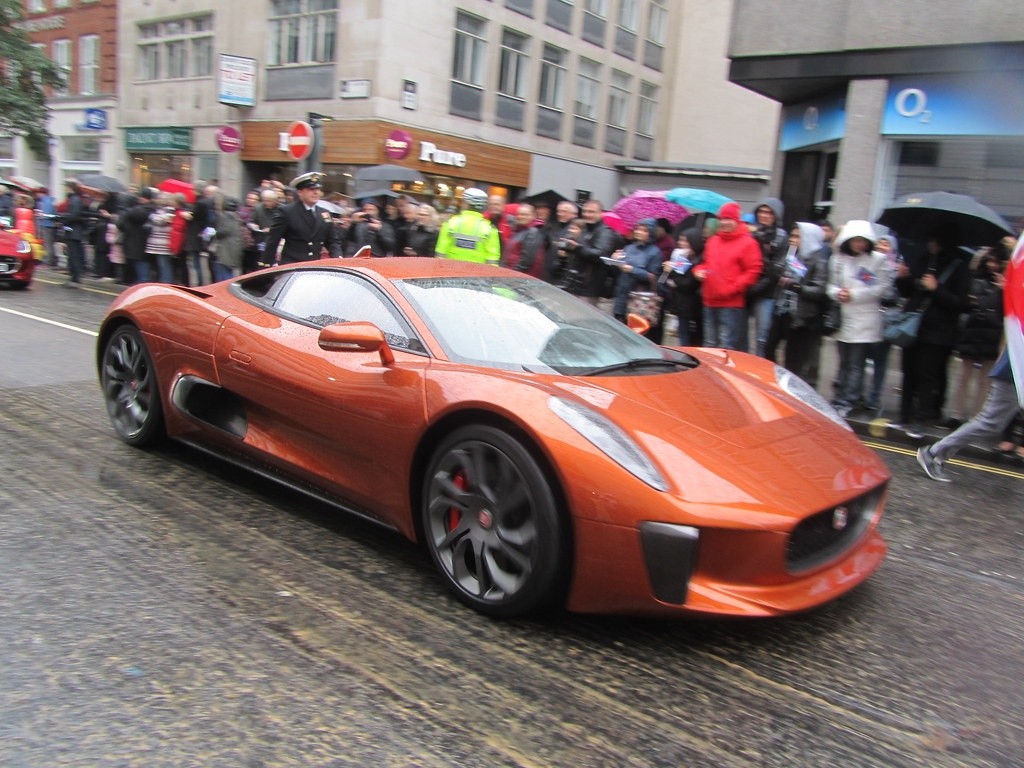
[0,223,32,288]
[95,246,893,623]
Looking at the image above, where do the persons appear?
[91,179,298,288]
[320,188,545,280]
[0,179,88,287]
[258,172,342,274]
[534,198,1024,484]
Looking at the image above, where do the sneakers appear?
[916,443,952,484]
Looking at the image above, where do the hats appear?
[60,178,79,191]
[636,216,657,238]
[135,186,153,201]
[462,187,489,210]
[716,203,742,224]
[288,171,327,189]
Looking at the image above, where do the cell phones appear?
[361,215,372,223]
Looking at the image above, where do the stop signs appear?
[287,121,316,160]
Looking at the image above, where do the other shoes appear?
[829,393,1024,463]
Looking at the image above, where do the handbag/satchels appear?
[626,290,665,330]
[880,313,924,348]
[817,302,843,336]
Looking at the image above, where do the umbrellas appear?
[664,186,735,216]
[352,164,429,184]
[75,174,129,194]
[156,178,197,203]
[521,189,582,216]
[611,190,693,230]
[601,212,633,235]
[873,187,1016,274]
[351,189,420,209]
